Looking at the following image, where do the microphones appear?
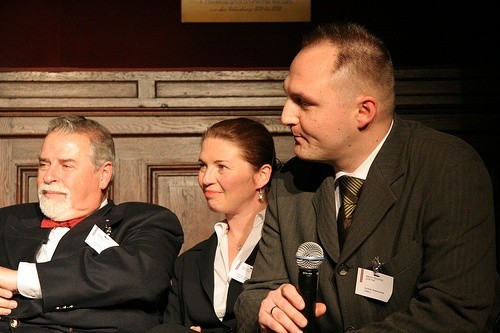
[296,241,324,333]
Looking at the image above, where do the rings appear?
[270,305,278,316]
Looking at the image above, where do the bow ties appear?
[40,215,88,230]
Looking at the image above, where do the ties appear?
[337,175,366,255]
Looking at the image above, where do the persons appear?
[0,114,184,333]
[164,118,276,333]
[234,24,496,333]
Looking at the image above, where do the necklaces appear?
[230,228,250,251]
